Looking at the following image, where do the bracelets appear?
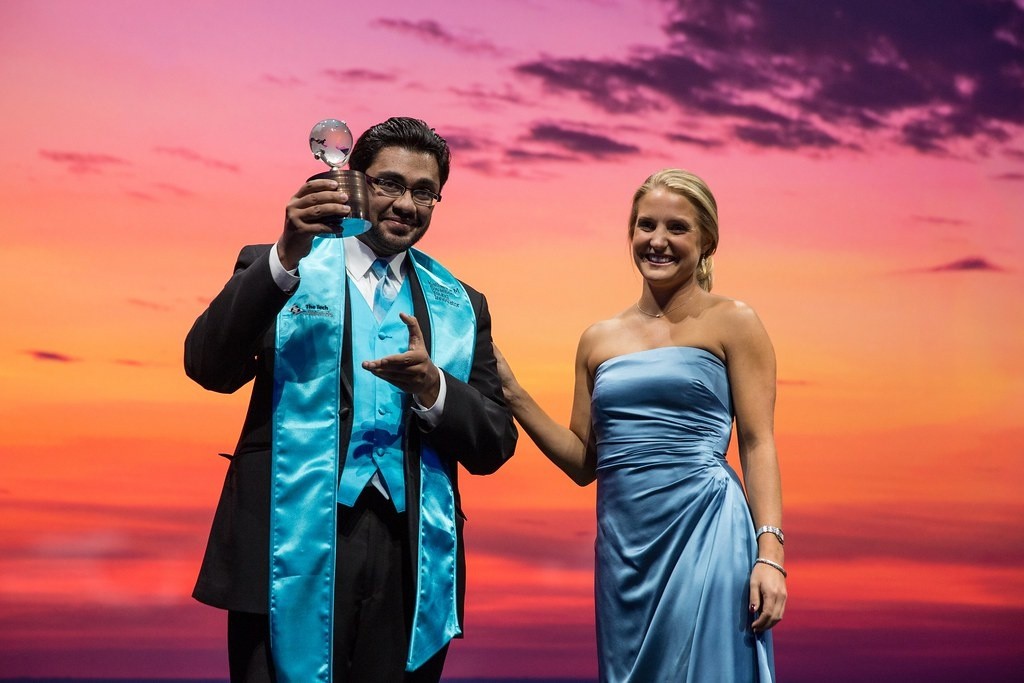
[755,558,787,578]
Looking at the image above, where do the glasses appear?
[364,172,442,206]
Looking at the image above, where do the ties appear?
[371,259,395,327]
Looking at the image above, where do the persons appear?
[489,168,788,683]
[183,115,520,683]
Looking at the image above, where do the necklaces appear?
[637,289,703,318]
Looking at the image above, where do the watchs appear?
[755,526,785,545]
